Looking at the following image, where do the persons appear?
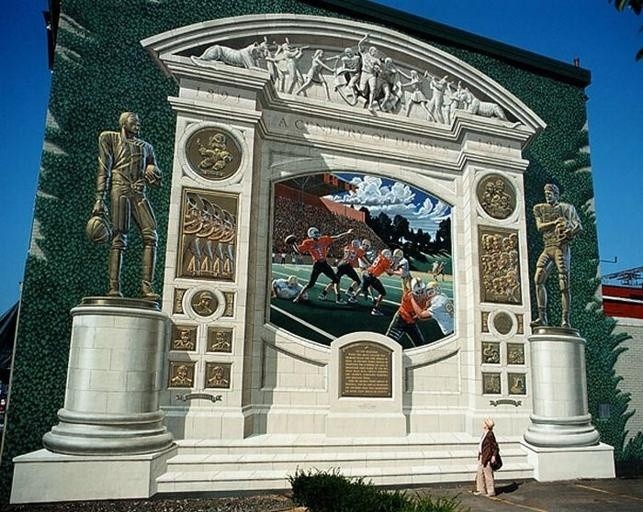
[272,195,455,348]
[473,417,500,497]
[532,183,583,327]
[258,32,464,125]
[93,112,162,302]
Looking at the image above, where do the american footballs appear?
[285,235,296,244]
[147,164,160,175]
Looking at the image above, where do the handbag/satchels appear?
[491,454,503,471]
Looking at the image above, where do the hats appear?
[481,417,494,429]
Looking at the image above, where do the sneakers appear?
[484,493,495,497]
[335,291,359,305]
[473,491,486,495]
[293,298,300,304]
[370,308,384,316]
[318,294,328,300]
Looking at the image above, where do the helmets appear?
[352,240,361,249]
[362,239,371,251]
[381,249,392,260]
[307,227,320,241]
[424,281,442,300]
[287,275,298,284]
[392,249,404,262]
[410,277,425,297]
